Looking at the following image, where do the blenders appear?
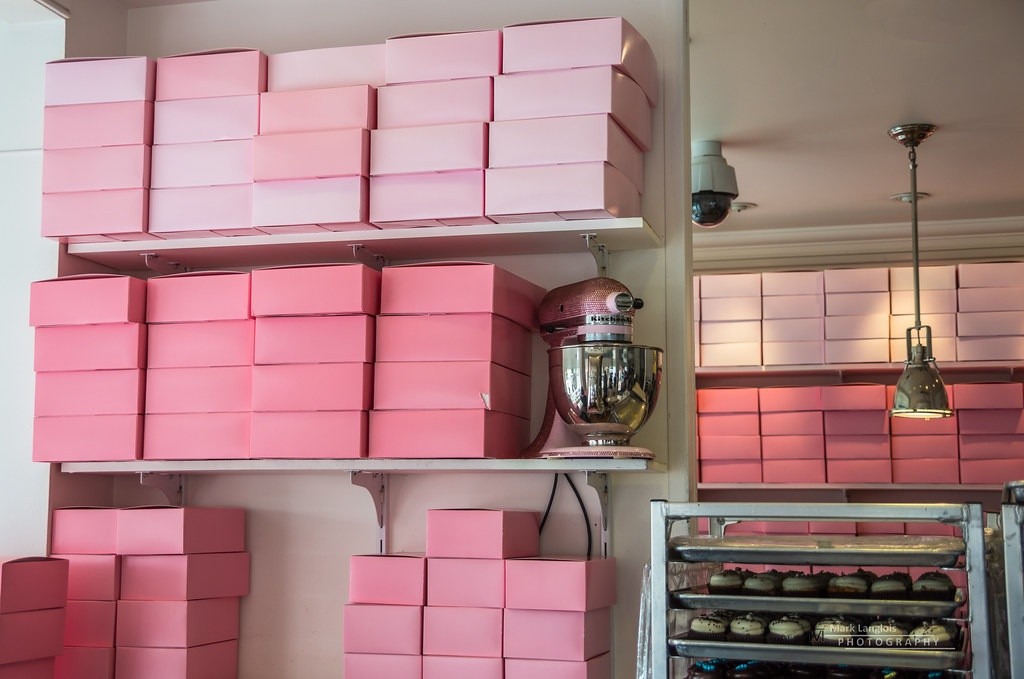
[519,274,664,461]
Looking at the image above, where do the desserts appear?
[682,567,959,679]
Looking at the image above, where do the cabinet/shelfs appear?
[697,360,1024,504]
[61,216,664,564]
[649,498,994,679]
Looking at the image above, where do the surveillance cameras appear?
[689,156,739,227]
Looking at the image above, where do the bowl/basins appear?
[546,343,664,446]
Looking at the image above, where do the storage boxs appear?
[0,504,251,679]
[692,262,1024,667]
[343,509,618,679]
[43,16,661,241]
[28,261,545,462]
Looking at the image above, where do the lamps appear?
[889,121,954,421]
